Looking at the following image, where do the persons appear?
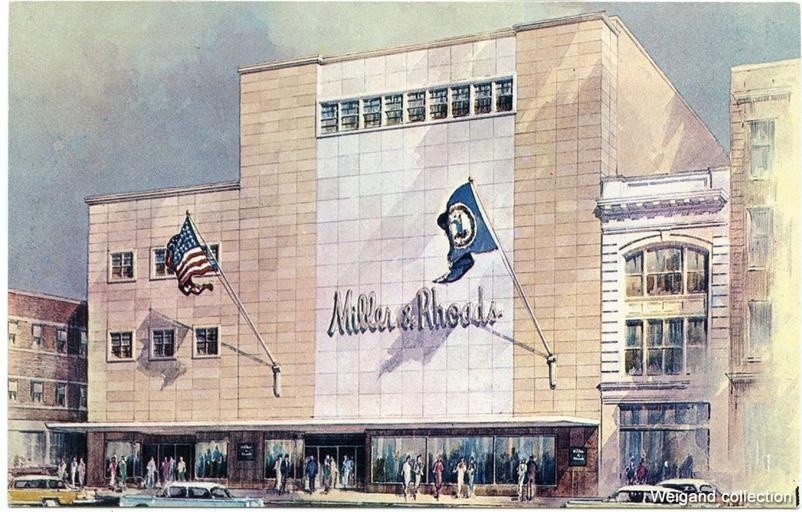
[273,453,354,494]
[636,458,648,484]
[499,447,538,501]
[55,443,220,492]
[625,456,636,485]
[397,454,476,499]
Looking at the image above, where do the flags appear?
[432,183,499,284]
[166,216,218,295]
